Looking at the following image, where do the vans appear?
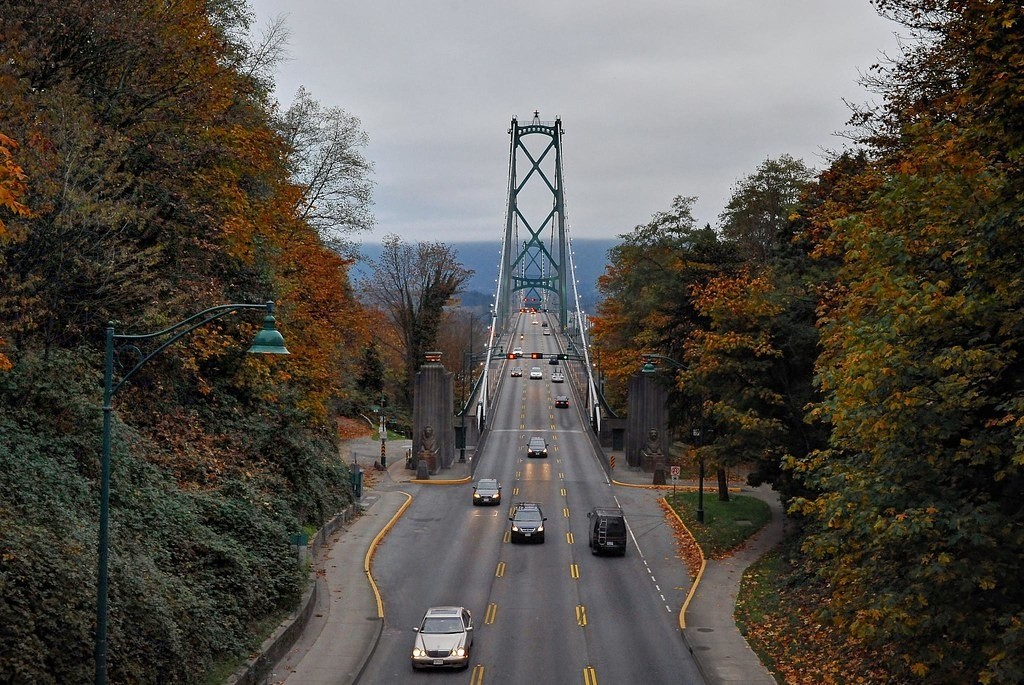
[586,506,628,556]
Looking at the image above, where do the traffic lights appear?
[532,353,543,359]
[558,354,568,360]
[505,354,517,359]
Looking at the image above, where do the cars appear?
[526,436,550,459]
[531,318,551,335]
[549,354,559,365]
[551,371,565,383]
[411,604,475,671]
[509,501,548,545]
[472,477,502,507]
[510,367,522,377]
[529,366,543,380]
[511,346,523,357]
[554,395,570,409]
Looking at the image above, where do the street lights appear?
[469,309,498,395]
[566,340,601,404]
[640,353,705,523]
[91,299,293,685]
[457,346,506,463]
